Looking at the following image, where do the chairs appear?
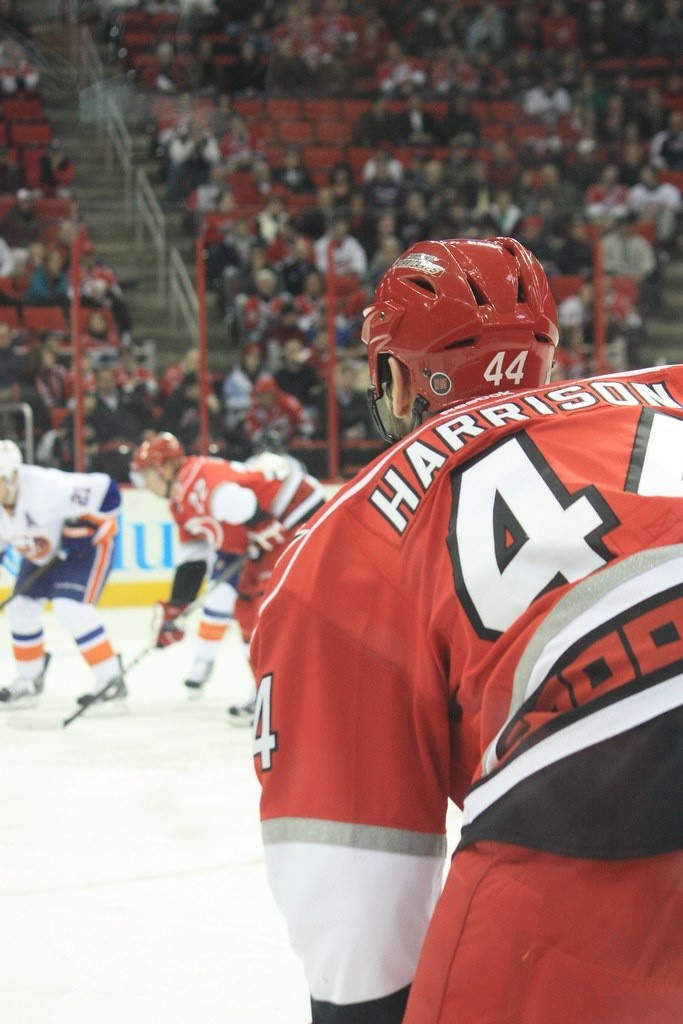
[0,0,683,472]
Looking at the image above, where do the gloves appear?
[158,602,184,644]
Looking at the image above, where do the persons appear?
[133,428,330,720]
[0,439,129,711]
[243,235,683,1024]
[0,0,682,468]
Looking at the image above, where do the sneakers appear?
[229,694,260,713]
[91,684,126,703]
[184,637,218,698]
[1,674,46,703]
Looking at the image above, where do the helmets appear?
[1,438,24,481]
[131,432,182,471]
[359,236,559,414]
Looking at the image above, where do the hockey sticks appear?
[4,549,250,732]
[0,554,60,610]
